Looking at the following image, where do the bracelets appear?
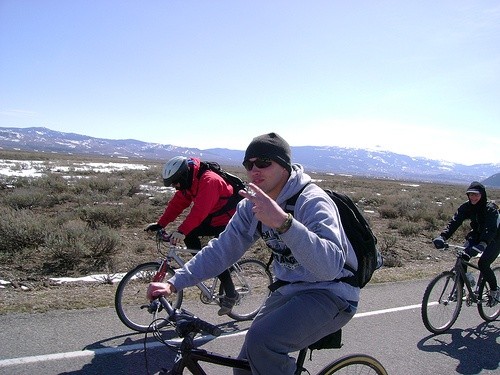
[276,213,292,232]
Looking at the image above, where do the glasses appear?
[242,157,273,172]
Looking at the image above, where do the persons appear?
[147,132,360,375]
[433,181,500,306]
[149,156,239,316]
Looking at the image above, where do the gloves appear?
[433,235,445,248]
[468,243,486,257]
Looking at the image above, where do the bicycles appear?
[131,244,137,249]
[420,245,500,333]
[150,293,389,375]
[115,222,274,331]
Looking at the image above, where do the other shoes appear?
[451,289,466,301]
[218,291,242,316]
[487,286,500,308]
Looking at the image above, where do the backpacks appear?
[285,181,384,289]
[197,160,247,214]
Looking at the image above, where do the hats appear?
[244,132,292,165]
[466,187,480,194]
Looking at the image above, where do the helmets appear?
[162,156,187,187]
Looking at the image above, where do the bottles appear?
[466,272,475,287]
